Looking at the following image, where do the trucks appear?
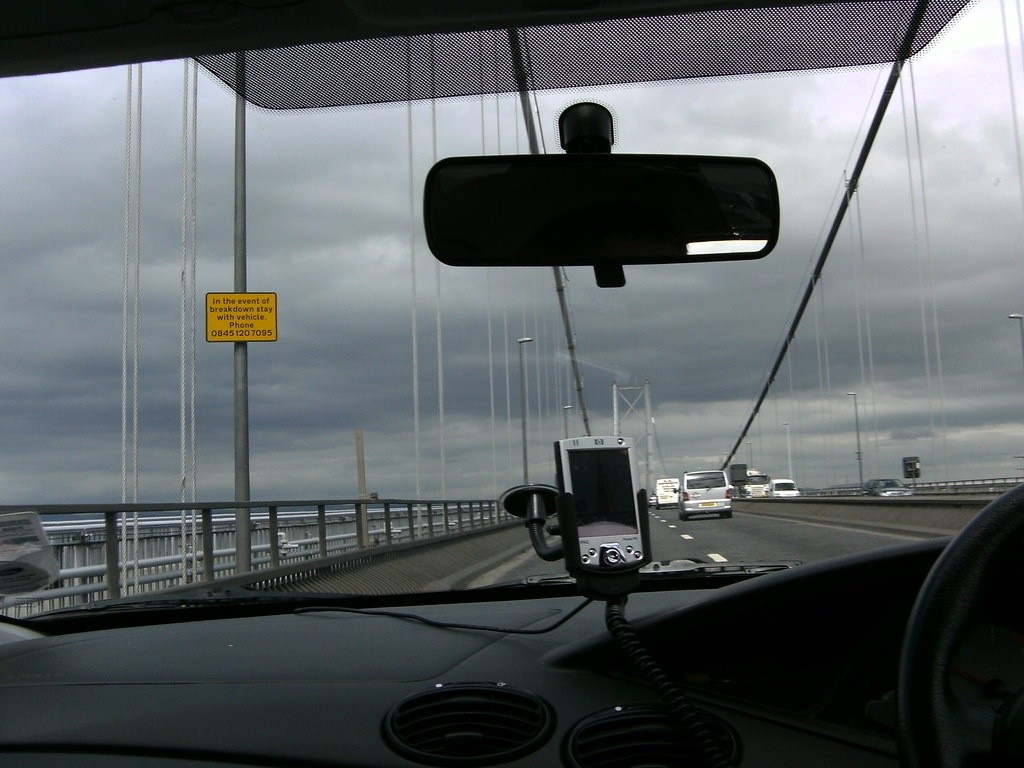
[729,470,770,499]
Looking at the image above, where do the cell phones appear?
[554,436,644,574]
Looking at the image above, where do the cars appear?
[649,493,656,508]
[862,478,913,498]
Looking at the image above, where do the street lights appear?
[846,393,864,490]
[781,422,793,481]
[517,337,534,486]
[1007,313,1024,364]
[563,405,573,439]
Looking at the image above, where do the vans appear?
[766,478,800,498]
[673,471,732,521]
[652,478,681,510]
